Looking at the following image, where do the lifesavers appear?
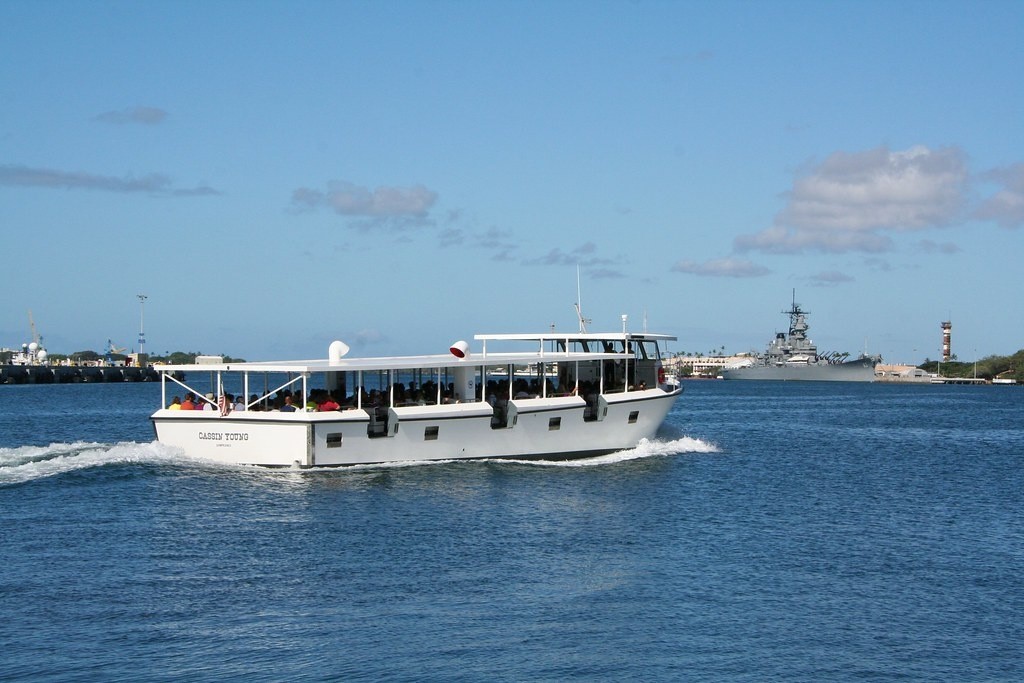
[658,367,666,384]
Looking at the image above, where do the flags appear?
[219,393,227,416]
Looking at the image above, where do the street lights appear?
[135,295,148,353]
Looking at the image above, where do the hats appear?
[238,398,244,402]
[205,393,215,401]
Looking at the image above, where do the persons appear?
[620,342,635,385]
[475,373,649,401]
[168,379,455,412]
[603,343,617,382]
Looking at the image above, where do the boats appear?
[721,288,881,383]
[149,263,684,472]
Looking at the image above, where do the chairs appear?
[272,392,573,413]
